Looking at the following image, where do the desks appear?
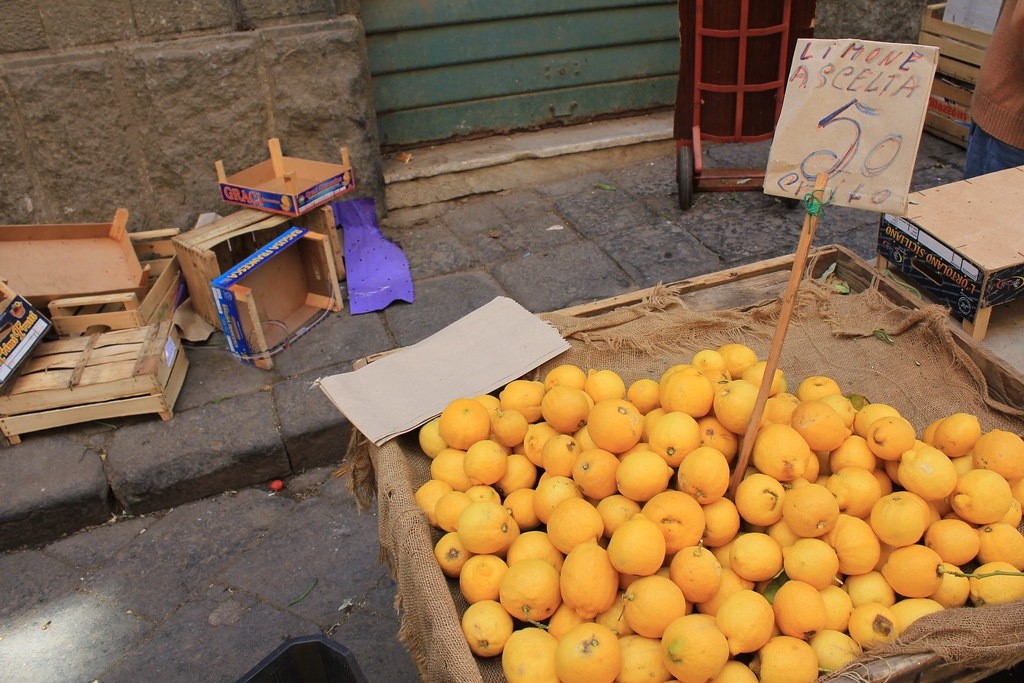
[875,165,1024,342]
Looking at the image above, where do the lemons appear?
[414,343,1024,683]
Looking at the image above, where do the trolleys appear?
[675,0,801,209]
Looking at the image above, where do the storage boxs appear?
[0,138,357,445]
[919,2,993,150]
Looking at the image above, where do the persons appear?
[962,0,1024,179]
